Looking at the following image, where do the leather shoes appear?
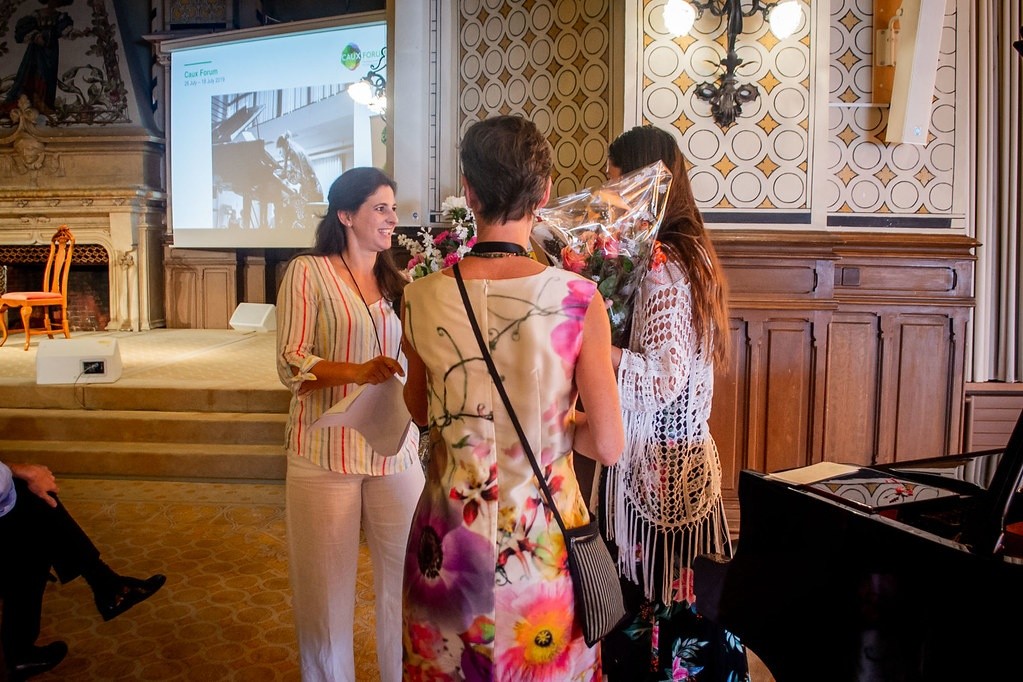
[7,640,67,682]
[98,574,167,621]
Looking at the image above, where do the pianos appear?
[690,409,1023,682]
[212,104,296,229]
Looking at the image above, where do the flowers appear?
[392,188,481,281]
[542,194,668,353]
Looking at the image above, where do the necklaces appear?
[462,252,534,259]
[339,251,403,362]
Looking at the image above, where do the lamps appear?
[662,0,800,129]
[345,71,385,111]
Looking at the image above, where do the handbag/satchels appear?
[565,510,630,648]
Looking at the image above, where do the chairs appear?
[0,223,76,352]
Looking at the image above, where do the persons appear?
[277,135,324,203]
[589,125,751,682]
[403,115,625,682]
[274,167,425,682]
[0,459,168,682]
